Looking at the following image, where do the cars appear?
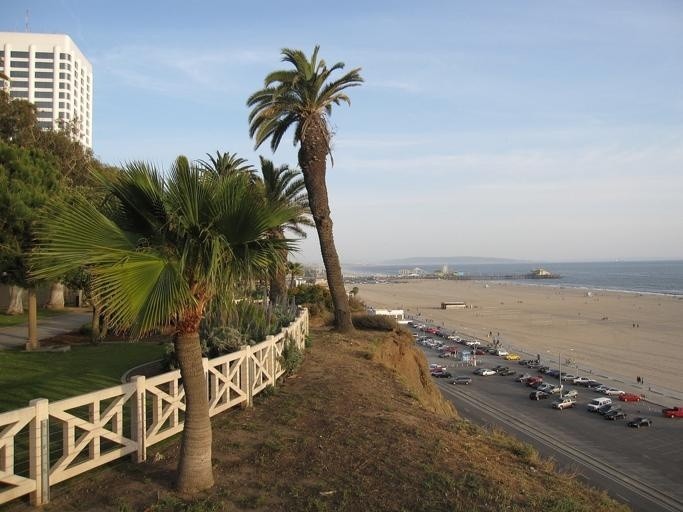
[408,322,683,428]
